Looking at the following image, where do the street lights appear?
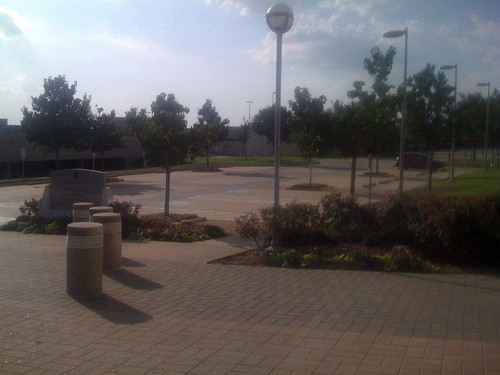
[475,81,491,158]
[382,27,408,195]
[246,101,253,121]
[265,2,295,256]
[440,63,458,184]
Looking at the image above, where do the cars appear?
[396,152,445,169]
